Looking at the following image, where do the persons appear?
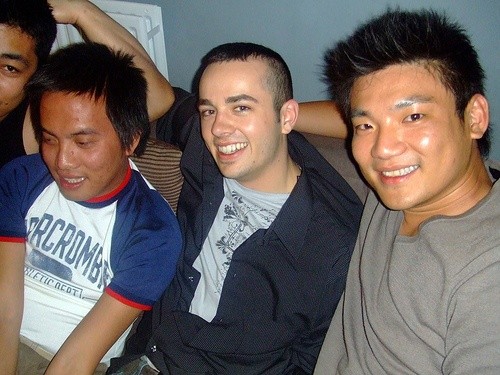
[0,42,182,375]
[0,0,175,166]
[106,42,364,375]
[292,6,500,375]
